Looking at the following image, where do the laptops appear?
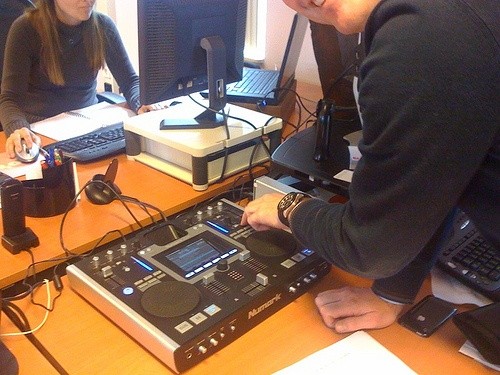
[199,12,309,105]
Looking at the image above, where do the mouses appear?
[14,140,39,163]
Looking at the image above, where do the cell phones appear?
[397,295,458,337]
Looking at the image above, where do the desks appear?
[0,81,500,375]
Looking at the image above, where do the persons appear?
[0,0,170,158]
[241,0,500,334]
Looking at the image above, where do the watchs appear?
[278,192,311,226]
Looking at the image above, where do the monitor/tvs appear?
[137,0,249,130]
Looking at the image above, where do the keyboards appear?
[43,122,126,162]
[434,210,500,301]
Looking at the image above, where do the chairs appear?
[0,0,127,132]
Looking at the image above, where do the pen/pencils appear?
[40,144,63,168]
[40,149,49,156]
